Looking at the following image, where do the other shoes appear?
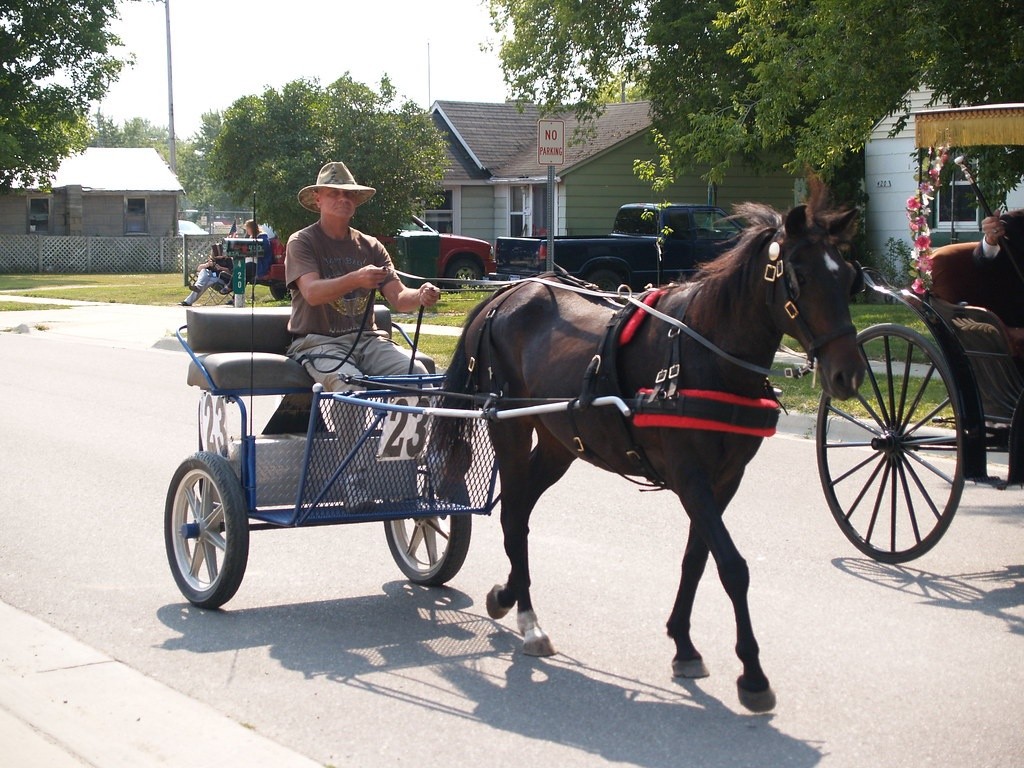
[188,284,202,295]
[225,299,234,304]
[179,301,193,306]
[220,287,232,295]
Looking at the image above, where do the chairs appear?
[187,270,234,306]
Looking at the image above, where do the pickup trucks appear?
[488,202,750,308]
[258,212,497,301]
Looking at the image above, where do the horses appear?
[437,202,867,712]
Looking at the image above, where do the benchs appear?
[176,307,435,395]
[926,240,1024,412]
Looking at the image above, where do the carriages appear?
[162,158,863,714]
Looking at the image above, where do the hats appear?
[298,160,376,214]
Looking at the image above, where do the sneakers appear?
[345,476,373,513]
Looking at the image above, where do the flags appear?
[229,220,236,235]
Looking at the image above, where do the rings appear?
[993,228,997,234]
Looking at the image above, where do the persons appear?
[220,220,272,304]
[284,162,472,515]
[972,208,1024,329]
[181,242,233,306]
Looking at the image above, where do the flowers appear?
[905,147,948,296]
